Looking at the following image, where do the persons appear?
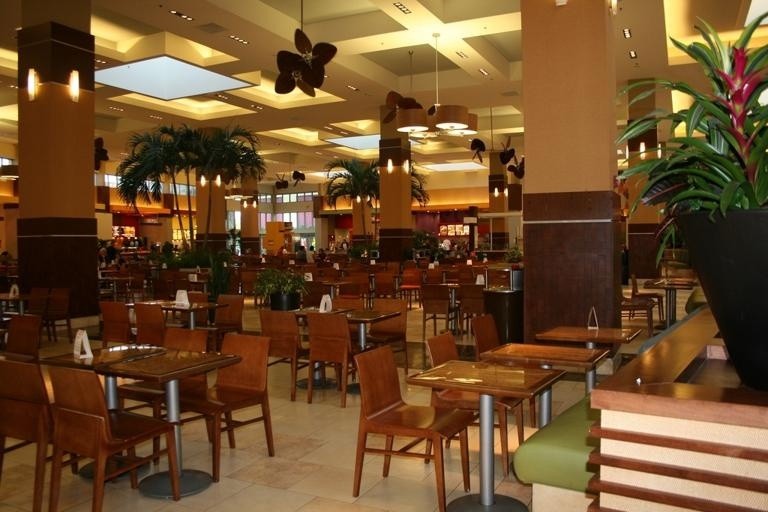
[442,236,453,250]
[341,239,349,253]
[294,245,307,265]
[306,246,316,264]
[96,227,130,268]
[0,251,9,265]
[318,248,327,264]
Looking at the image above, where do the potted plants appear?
[614,9,768,392]
